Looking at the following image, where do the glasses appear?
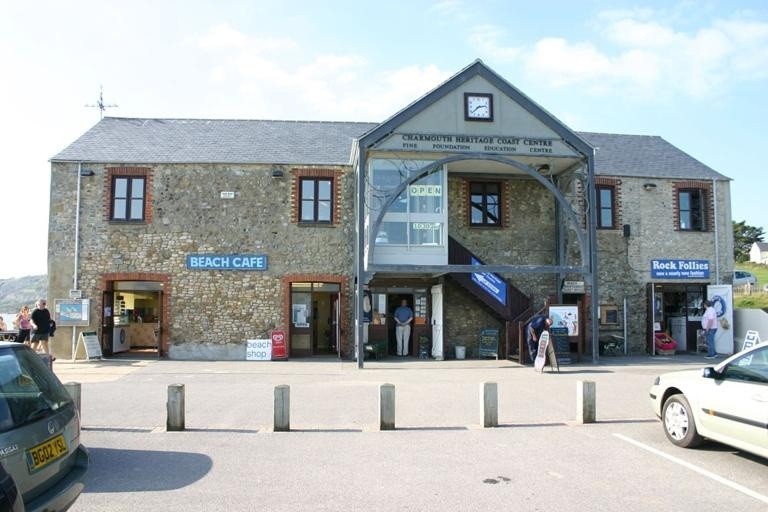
[42,303,46,305]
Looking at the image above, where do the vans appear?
[0,340,100,512]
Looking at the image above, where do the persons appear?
[394,300,414,357]
[0,317,7,331]
[525,315,553,362]
[29,298,56,361]
[701,300,718,359]
[15,306,32,345]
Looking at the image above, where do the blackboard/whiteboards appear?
[550,328,572,365]
[479,327,500,357]
[81,331,103,359]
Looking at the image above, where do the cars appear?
[732,269,757,288]
[646,338,768,462]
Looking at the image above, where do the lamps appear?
[536,165,549,175]
[644,183,656,190]
[81,170,93,176]
[272,170,284,178]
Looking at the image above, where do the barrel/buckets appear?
[455,346,467,359]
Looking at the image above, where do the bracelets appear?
[400,323,401,326]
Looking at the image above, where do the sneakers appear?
[713,353,719,358]
[703,355,715,359]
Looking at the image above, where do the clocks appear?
[464,93,494,121]
